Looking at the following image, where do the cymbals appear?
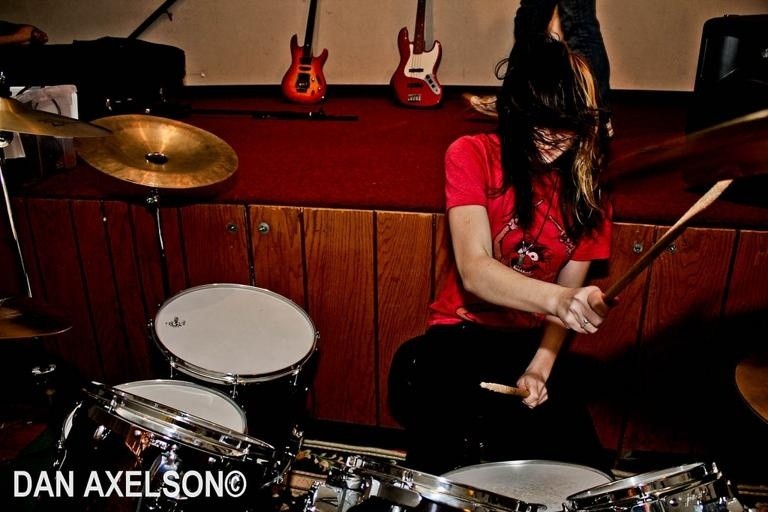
[734,352,768,424]
[74,115,237,188]
[0,296,73,338]
[0,98,112,139]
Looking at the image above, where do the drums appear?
[563,461,755,510]
[56,378,271,510]
[439,460,613,511]
[148,283,320,456]
[301,452,547,511]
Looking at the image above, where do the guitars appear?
[390,2,442,109]
[281,1,329,105]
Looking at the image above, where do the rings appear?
[580,319,590,328]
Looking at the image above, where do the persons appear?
[0,24,49,46]
[462,0,615,138]
[386,30,617,480]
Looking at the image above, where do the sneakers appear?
[595,116,614,138]
[470,92,499,119]
